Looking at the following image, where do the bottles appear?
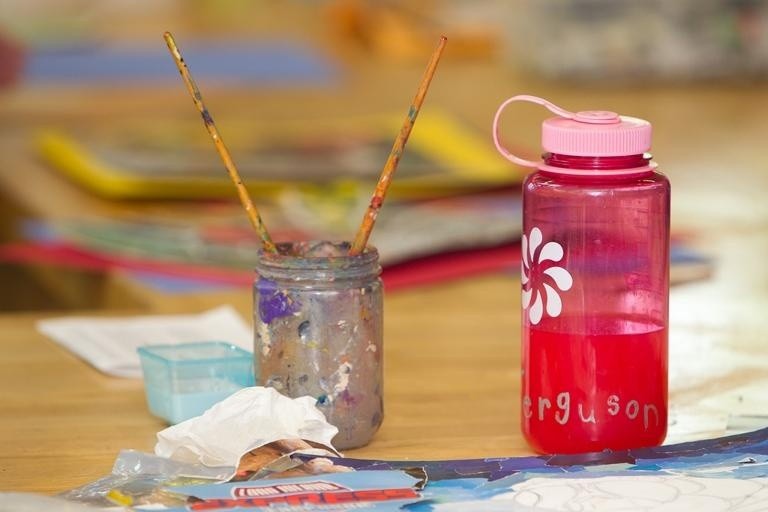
[489,96,672,456]
